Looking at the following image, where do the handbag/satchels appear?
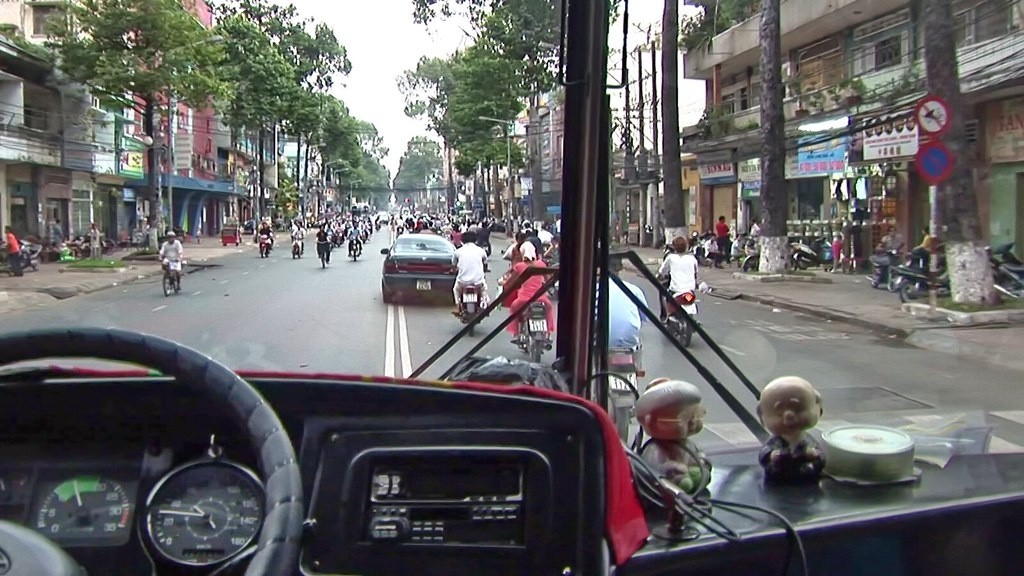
[169,262,181,271]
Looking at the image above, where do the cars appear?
[380,230,459,303]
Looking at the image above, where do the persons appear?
[4,225,25,276]
[450,231,490,316]
[832,222,860,273]
[875,226,931,253]
[497,216,560,344]
[158,231,184,291]
[258,219,274,253]
[652,236,699,324]
[595,255,650,372]
[759,375,823,488]
[52,218,100,261]
[703,216,763,269]
[634,377,711,494]
[290,213,382,265]
[196,229,201,243]
[390,209,502,242]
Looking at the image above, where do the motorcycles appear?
[450,265,490,337]
[497,274,554,364]
[220,221,243,245]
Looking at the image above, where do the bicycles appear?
[316,241,332,269]
[158,259,185,297]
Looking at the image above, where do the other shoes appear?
[177,285,180,290]
[510,335,520,343]
[451,310,459,315]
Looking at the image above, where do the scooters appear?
[290,236,305,259]
[864,240,1024,305]
[654,273,703,348]
[258,234,274,258]
[0,231,117,277]
[589,348,646,444]
[324,211,490,262]
[689,231,833,273]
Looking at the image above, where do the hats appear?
[167,231,175,237]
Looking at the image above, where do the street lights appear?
[168,34,223,232]
[477,116,512,238]
[324,159,343,213]
[333,166,350,211]
[303,143,326,230]
[349,179,362,211]
